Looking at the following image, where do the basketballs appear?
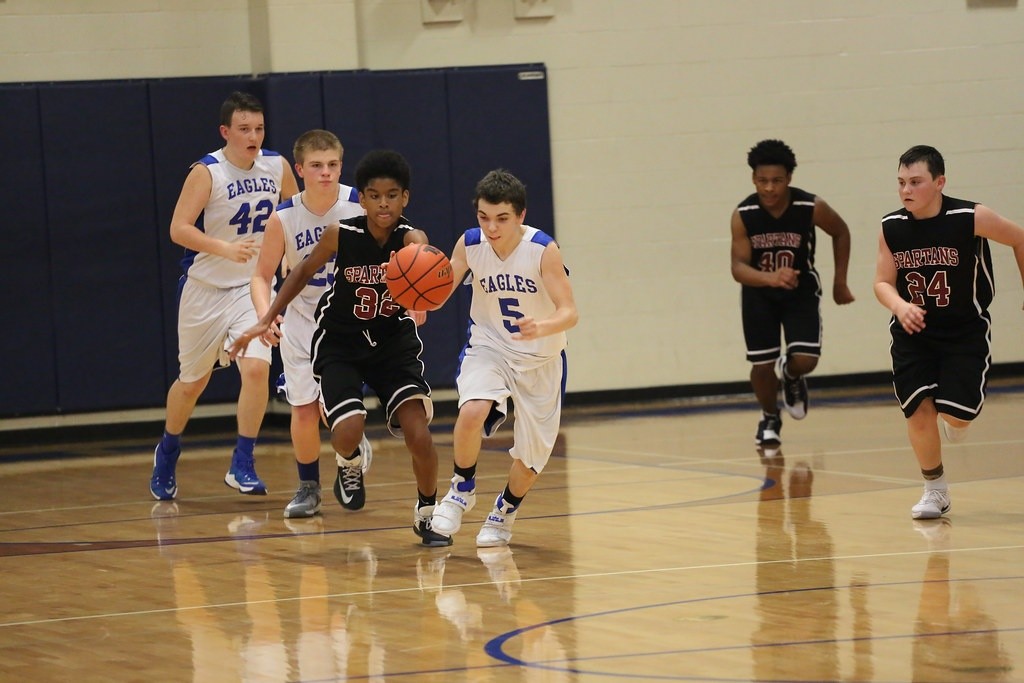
[385,241,455,314]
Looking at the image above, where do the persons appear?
[730,139,855,445]
[250,130,373,519]
[149,90,300,500]
[873,144,1024,520]
[380,170,579,548]
[226,147,439,537]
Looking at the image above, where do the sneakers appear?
[333,448,366,513]
[911,486,951,519]
[756,408,782,447]
[476,495,517,547]
[775,355,809,420]
[945,418,972,444]
[223,450,267,496]
[284,480,327,519]
[360,431,373,475]
[413,500,453,547]
[150,439,181,500]
[431,472,477,535]
